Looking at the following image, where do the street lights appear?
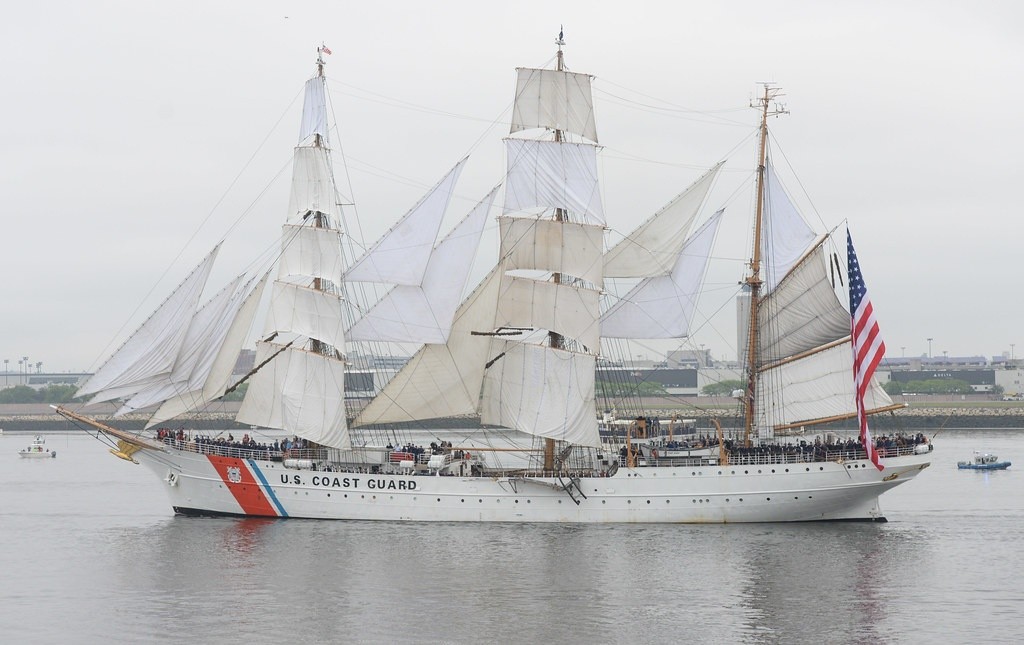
[18,360,24,384]
[926,338,933,359]
[4,359,9,386]
[1010,344,1015,359]
[23,355,28,384]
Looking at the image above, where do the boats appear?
[18,435,57,459]
[957,451,1012,470]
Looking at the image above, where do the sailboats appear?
[45,24,958,524]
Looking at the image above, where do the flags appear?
[321,44,332,55]
[846,228,887,472]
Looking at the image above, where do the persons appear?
[153,427,327,462]
[618,415,933,469]
[494,469,605,480]
[378,469,384,474]
[323,465,332,471]
[386,441,486,462]
